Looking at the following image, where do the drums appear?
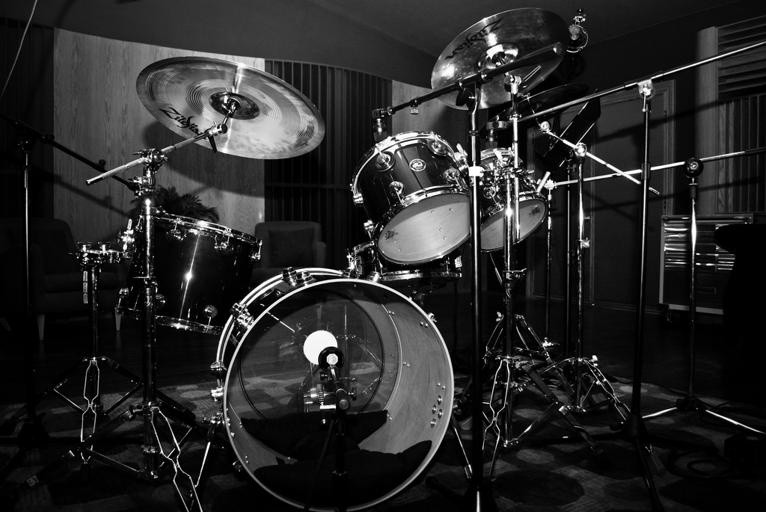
[210,263,455,512]
[469,148,547,255]
[347,240,462,288]
[348,129,476,266]
[136,206,262,337]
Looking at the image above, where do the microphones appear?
[371,110,388,143]
[324,365,351,411]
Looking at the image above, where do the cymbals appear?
[429,8,568,111]
[137,56,325,163]
[466,81,585,154]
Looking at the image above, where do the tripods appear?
[23,114,245,512]
[0,245,197,438]
[449,39,766,512]
